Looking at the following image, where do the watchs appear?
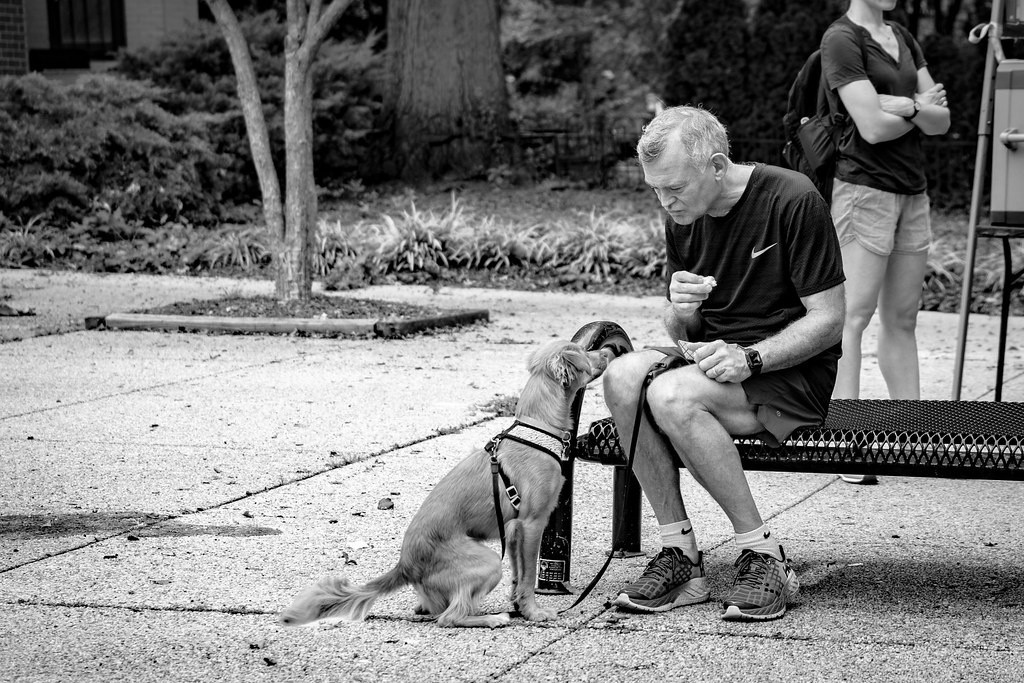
[905,101,921,120]
[742,347,764,375]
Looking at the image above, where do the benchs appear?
[532,321,1024,595]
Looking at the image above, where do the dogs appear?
[281,339,609,629]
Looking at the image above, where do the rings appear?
[713,368,718,377]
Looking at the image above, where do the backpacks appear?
[783,19,918,193]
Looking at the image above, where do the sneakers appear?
[611,547,709,611]
[837,472,879,484]
[720,548,801,620]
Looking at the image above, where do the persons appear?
[815,0,949,486]
[602,103,847,622]
